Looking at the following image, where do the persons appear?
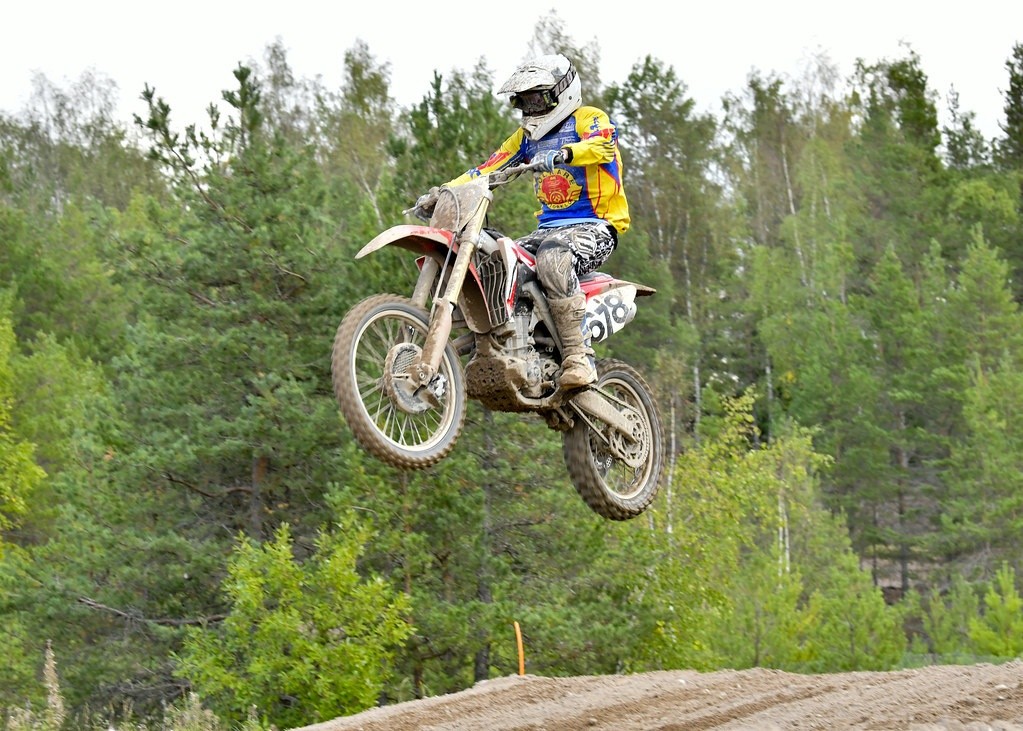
[413,54,630,388]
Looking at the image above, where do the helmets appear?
[497,54,582,141]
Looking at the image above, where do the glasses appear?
[510,91,554,114]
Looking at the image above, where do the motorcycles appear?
[330,156,665,523]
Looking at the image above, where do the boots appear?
[546,295,599,390]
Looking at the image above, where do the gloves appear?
[414,194,432,222]
[530,150,565,171]
